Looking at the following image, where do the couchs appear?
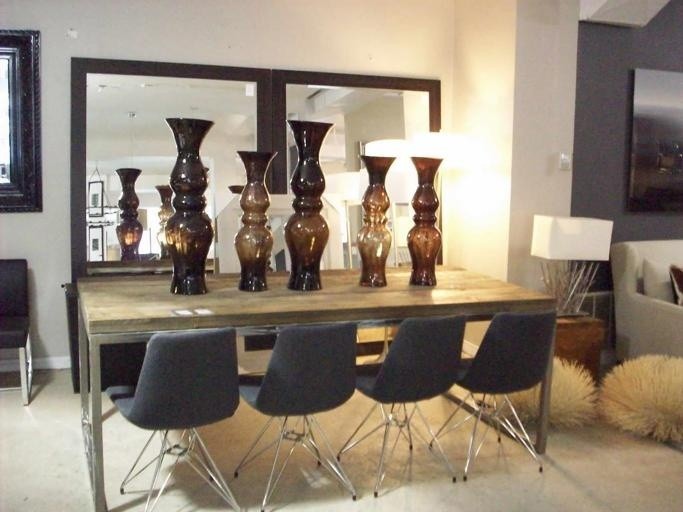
[610,237,683,365]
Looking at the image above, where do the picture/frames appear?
[628,67,682,213]
[88,226,104,261]
[88,181,104,217]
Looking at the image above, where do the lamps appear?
[327,172,365,270]
[529,214,614,317]
[365,137,411,269]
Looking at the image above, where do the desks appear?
[553,316,604,383]
[71,263,556,512]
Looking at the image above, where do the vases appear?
[285,119,334,293]
[356,154,398,287]
[154,185,176,263]
[409,156,444,286]
[163,117,216,297]
[236,150,281,293]
[115,168,144,264]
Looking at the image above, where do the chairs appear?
[337,313,465,496]
[428,309,557,479]
[0,259,33,406]
[236,321,359,512]
[107,325,241,511]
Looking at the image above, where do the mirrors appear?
[271,68,442,271]
[70,56,271,280]
[0,30,43,213]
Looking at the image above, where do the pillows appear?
[668,263,683,306]
[641,257,675,304]
